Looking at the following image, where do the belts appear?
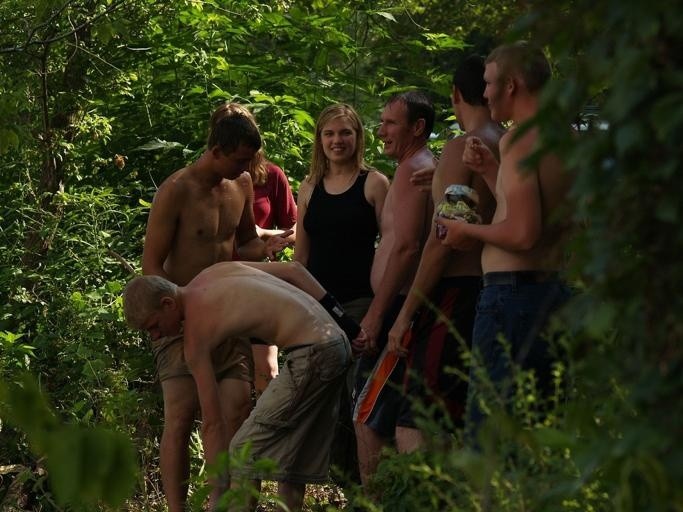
[484,270,560,285]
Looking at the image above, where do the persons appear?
[138,113,289,512]
[432,39,585,456]
[122,260,368,512]
[294,102,390,492]
[356,90,442,503]
[211,103,299,404]
[386,51,510,456]
